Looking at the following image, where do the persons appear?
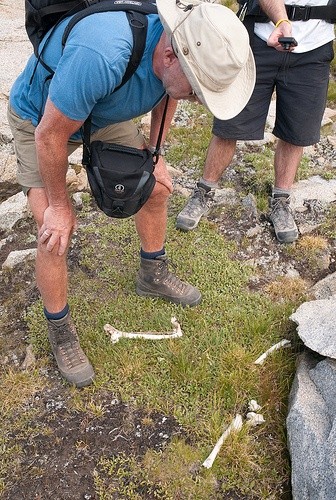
[6,0,256,388]
[175,0,336,243]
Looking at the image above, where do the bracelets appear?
[149,145,162,155]
[275,18,288,28]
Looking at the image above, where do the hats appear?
[156,0,258,122]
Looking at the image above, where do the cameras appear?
[278,37,298,50]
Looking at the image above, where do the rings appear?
[44,232,48,236]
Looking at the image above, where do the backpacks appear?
[22,0,160,93]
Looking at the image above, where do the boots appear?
[43,314,94,386]
[137,251,202,305]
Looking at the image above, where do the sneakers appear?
[267,194,299,243]
[175,180,215,230]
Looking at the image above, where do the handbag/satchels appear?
[84,141,155,219]
